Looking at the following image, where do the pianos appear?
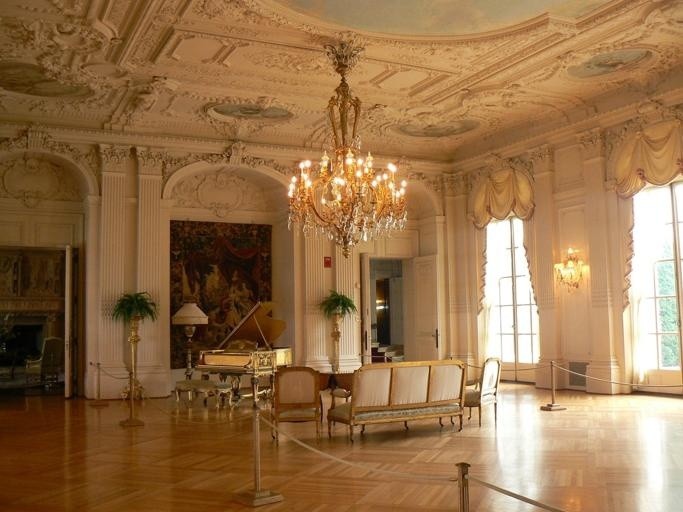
[196,300,293,410]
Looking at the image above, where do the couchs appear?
[327,359,466,442]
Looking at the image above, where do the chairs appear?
[463,355,502,427]
[270,367,324,448]
[24,335,63,387]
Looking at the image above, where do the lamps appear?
[553,247,590,294]
[286,38,407,259]
[172,303,209,377]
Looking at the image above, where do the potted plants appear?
[111,291,160,326]
[318,289,358,321]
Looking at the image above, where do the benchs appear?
[175,380,233,408]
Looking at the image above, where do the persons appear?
[193,279,255,343]
